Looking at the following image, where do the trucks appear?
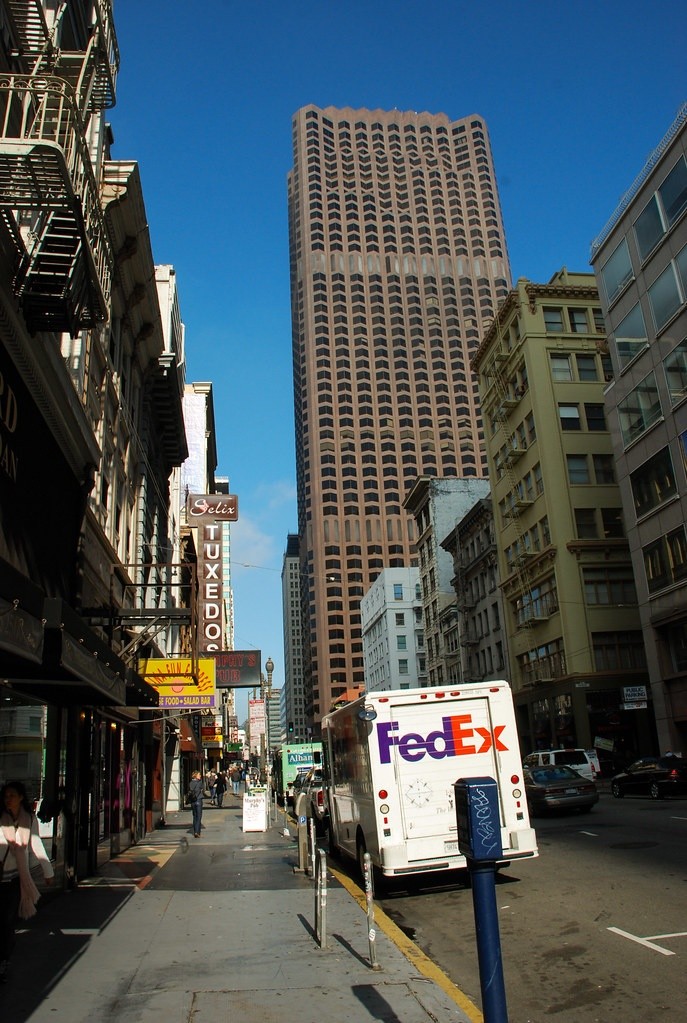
[314,679,539,891]
[272,742,323,805]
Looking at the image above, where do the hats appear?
[216,772,221,775]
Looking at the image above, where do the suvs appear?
[523,749,597,784]
[610,754,687,800]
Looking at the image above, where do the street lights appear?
[252,659,274,786]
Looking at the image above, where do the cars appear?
[287,764,323,836]
[522,764,601,815]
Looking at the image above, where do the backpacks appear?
[208,772,216,787]
[227,768,233,777]
[185,781,201,805]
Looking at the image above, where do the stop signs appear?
[454,777,508,1022]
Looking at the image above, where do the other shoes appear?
[0,959,12,982]
[219,805,224,808]
[194,832,200,838]
[211,800,215,805]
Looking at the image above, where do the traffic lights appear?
[289,722,294,732]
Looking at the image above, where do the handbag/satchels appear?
[212,784,218,788]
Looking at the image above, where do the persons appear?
[229,767,242,795]
[213,773,227,808]
[188,769,205,838]
[0,779,55,964]
[207,768,218,804]
